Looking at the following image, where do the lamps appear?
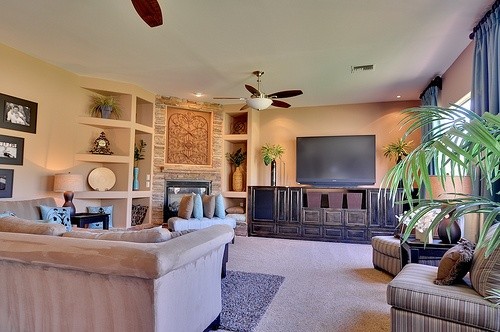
[54,171,83,215]
[246,95,274,111]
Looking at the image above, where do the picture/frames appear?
[0,93,39,198]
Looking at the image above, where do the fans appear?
[214,71,303,111]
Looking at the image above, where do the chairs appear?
[372,236,401,275]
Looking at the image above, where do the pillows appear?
[86,206,112,229]
[434,238,476,286]
[39,205,71,231]
[178,192,225,220]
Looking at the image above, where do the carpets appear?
[220,270,285,332]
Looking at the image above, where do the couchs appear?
[388,223,500,332]
[0,198,235,332]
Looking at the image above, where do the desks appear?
[400,234,456,271]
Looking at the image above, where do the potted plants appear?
[89,94,121,120]
[226,144,285,191]
[383,139,416,188]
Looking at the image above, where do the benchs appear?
[169,217,236,244]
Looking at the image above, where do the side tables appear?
[70,213,111,230]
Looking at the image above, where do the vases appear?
[134,140,146,190]
[438,206,461,244]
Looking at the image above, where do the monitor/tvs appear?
[296,135,376,187]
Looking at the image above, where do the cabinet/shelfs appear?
[80,117,153,231]
[225,134,247,199]
[247,186,418,244]
